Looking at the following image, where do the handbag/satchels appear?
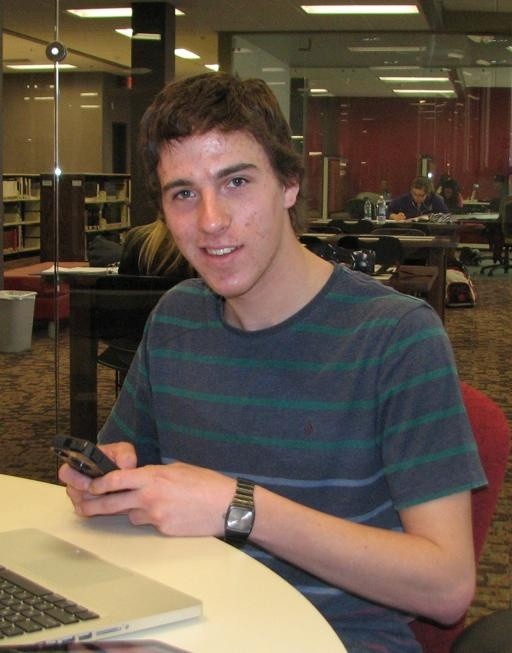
[443,261,476,308]
[320,244,376,273]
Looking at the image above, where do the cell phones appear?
[47,434,131,495]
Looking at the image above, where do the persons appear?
[484,174,507,243]
[437,180,465,262]
[107,200,200,366]
[57,71,488,653]
[386,176,449,300]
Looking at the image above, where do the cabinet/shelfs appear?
[0,172,132,260]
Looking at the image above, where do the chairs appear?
[68,272,191,447]
[408,382,511,652]
[301,195,510,278]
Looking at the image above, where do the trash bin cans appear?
[0,289,38,353]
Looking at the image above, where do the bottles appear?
[376,194,386,224]
[363,197,371,221]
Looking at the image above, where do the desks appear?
[2,259,91,339]
[1,465,349,653]
[343,263,440,299]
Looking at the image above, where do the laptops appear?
[0,528,203,653]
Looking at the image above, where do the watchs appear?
[224,477,257,549]
